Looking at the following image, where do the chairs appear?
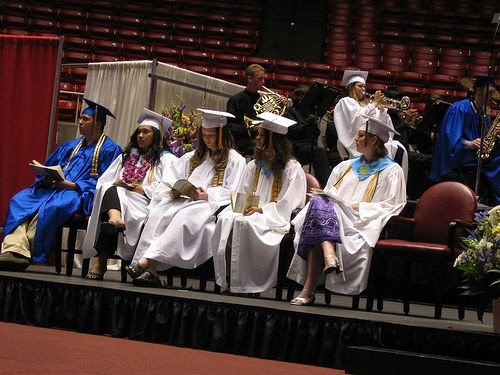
[56,174,485,320]
[0,0,500,124]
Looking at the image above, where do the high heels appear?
[322,257,343,274]
[290,294,317,307]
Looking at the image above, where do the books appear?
[163,179,199,197]
[231,192,260,214]
[27,159,65,181]
[307,188,335,199]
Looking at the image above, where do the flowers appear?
[163,95,203,158]
[453,204,500,297]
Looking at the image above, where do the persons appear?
[429,77,500,206]
[80,62,406,304]
[0,98,123,270]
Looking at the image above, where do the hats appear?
[81,97,117,136]
[136,108,173,146]
[471,74,500,88]
[357,113,400,147]
[341,69,368,89]
[196,108,236,150]
[257,111,297,158]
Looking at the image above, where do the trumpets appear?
[362,91,411,112]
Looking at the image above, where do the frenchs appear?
[243,86,287,137]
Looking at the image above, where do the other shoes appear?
[237,293,246,297]
[248,293,260,298]
[0,252,30,271]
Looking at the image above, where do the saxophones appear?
[475,98,500,160]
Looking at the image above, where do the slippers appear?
[101,220,126,233]
[86,263,107,280]
[125,262,143,279]
[132,271,161,287]
